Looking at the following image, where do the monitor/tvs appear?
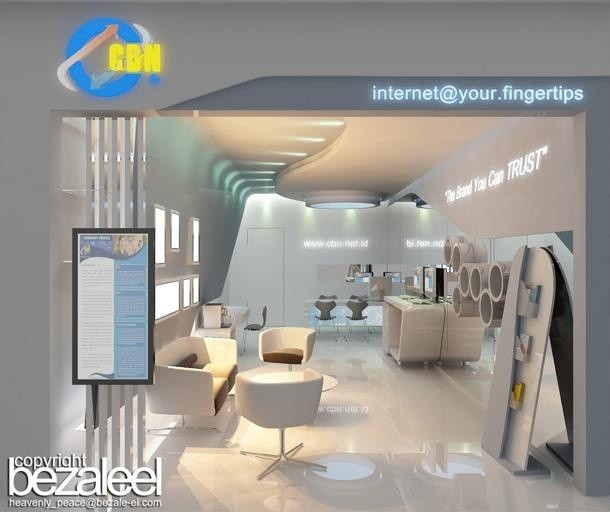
[424,267,439,302]
[439,268,448,297]
[413,267,424,298]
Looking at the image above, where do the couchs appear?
[145,337,240,435]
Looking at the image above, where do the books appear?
[519,332,533,355]
[511,380,526,408]
[525,283,542,319]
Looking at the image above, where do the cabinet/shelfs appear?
[383,294,445,365]
[189,306,234,340]
[438,298,486,365]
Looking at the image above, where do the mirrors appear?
[435,230,574,478]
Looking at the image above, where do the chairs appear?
[233,371,323,480]
[303,295,383,343]
[259,326,315,370]
[244,306,267,352]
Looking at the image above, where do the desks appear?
[227,303,251,354]
